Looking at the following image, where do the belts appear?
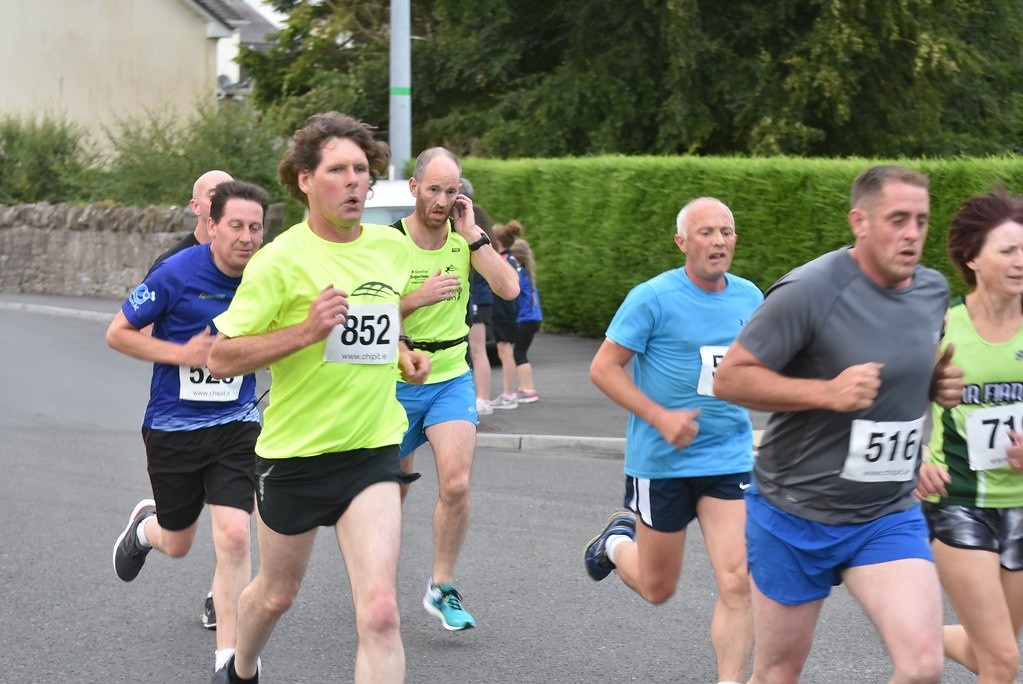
[410,339,471,354]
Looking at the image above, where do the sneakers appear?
[489,394,519,409]
[582,512,637,583]
[201,597,218,629]
[112,498,158,584]
[422,576,477,633]
[513,389,539,404]
[477,398,493,416]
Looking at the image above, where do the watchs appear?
[465,232,492,252]
[398,335,415,351]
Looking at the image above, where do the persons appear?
[489,224,522,412]
[141,169,235,282]
[214,113,428,684]
[511,240,546,403]
[912,187,1023,684]
[104,178,272,684]
[380,147,521,635]
[582,196,766,682]
[463,205,500,417]
[710,164,968,684]
